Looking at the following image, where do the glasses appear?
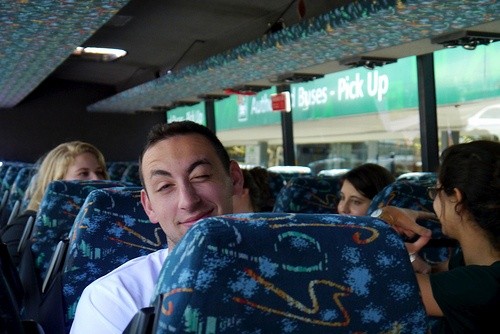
[428,186,444,200]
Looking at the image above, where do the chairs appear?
[0,160,464,334]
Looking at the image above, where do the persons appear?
[27,141,108,212]
[69,121,433,334]
[232,167,283,213]
[338,164,393,216]
[405,142,500,334]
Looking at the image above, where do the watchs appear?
[371,209,383,219]
